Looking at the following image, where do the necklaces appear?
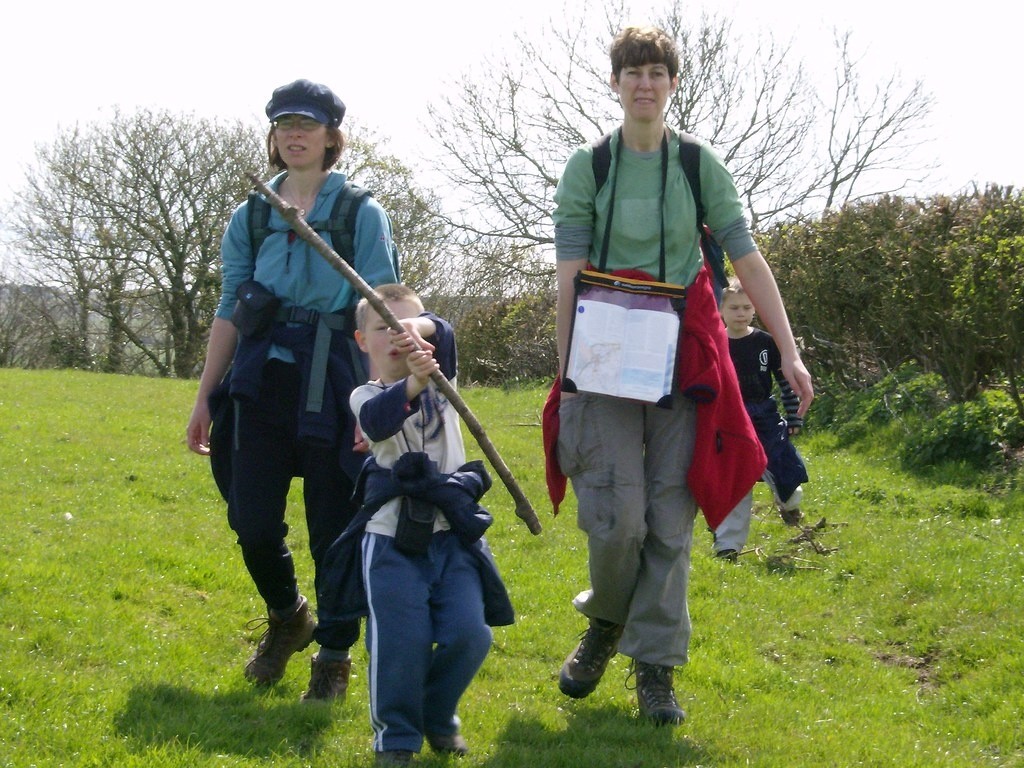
[284,181,321,216]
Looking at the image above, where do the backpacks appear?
[592,132,730,310]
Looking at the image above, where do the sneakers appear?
[717,549,737,564]
[300,651,352,702]
[244,594,318,686]
[559,617,625,699]
[625,660,684,724]
[782,508,799,526]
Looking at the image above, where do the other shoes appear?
[427,731,466,756]
[376,749,412,768]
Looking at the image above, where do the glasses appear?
[277,119,329,130]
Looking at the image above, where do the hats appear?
[266,79,346,127]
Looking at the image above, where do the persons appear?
[350,286,516,768]
[187,78,395,700]
[557,28,815,724]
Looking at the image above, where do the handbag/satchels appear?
[559,268,687,409]
